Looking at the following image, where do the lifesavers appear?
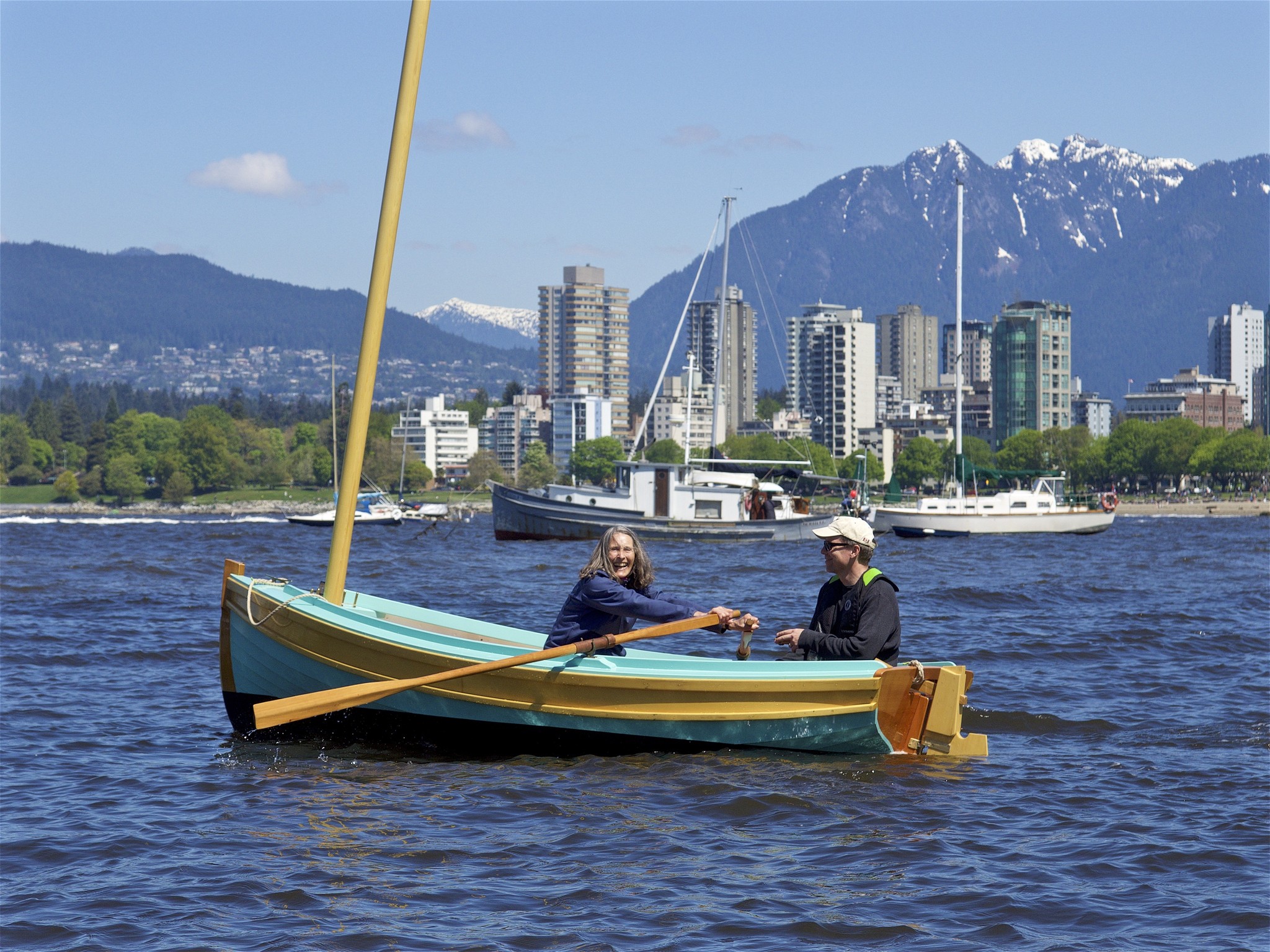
[1102,492,1117,510]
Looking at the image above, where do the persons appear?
[977,486,1009,496]
[839,491,853,516]
[760,491,776,520]
[774,514,903,670]
[544,524,761,657]
[1078,482,1269,514]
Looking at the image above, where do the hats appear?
[811,516,876,550]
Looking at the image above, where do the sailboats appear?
[392,397,451,520]
[218,2,990,761]
[485,195,870,543]
[283,354,403,524]
[835,181,1119,534]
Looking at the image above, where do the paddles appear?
[737,617,754,660]
[254,609,741,731]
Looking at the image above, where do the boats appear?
[890,523,971,539]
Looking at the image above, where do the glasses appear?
[824,541,850,551]
